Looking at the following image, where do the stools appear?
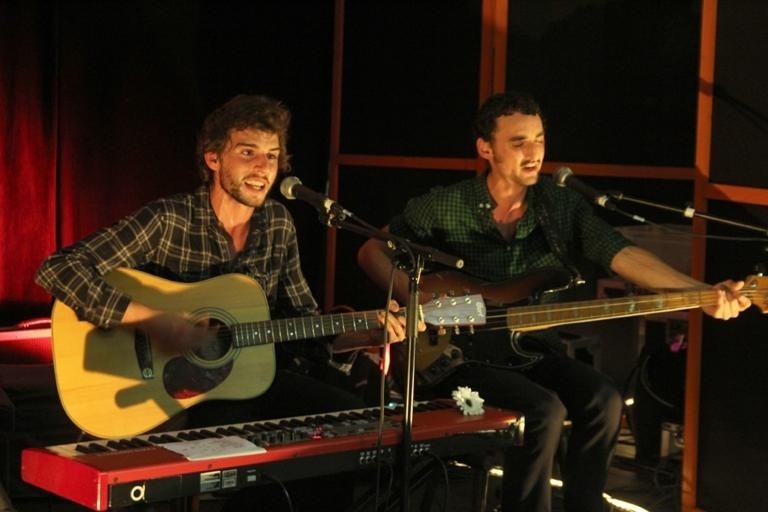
[417,413,571,511]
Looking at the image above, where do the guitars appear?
[393,264,768,390]
[51,268,487,440]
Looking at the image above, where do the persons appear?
[355,92,755,511]
[33,93,428,511]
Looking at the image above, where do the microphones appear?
[278,176,344,225]
[551,165,619,213]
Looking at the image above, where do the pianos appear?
[20,398,520,511]
